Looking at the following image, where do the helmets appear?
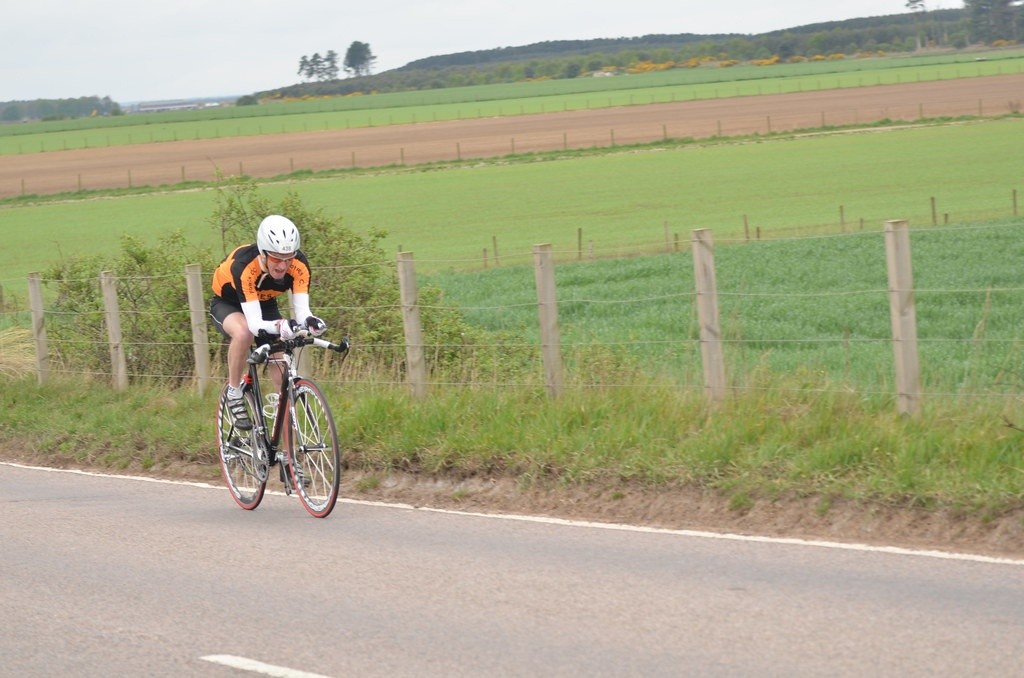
[256,215,300,258]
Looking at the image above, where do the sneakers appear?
[224,396,253,431]
[280,451,310,489]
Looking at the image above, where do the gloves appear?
[305,316,328,337]
[279,319,308,342]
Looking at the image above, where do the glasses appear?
[267,255,293,263]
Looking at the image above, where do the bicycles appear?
[216,325,349,519]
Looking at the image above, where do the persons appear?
[210,214,327,489]
